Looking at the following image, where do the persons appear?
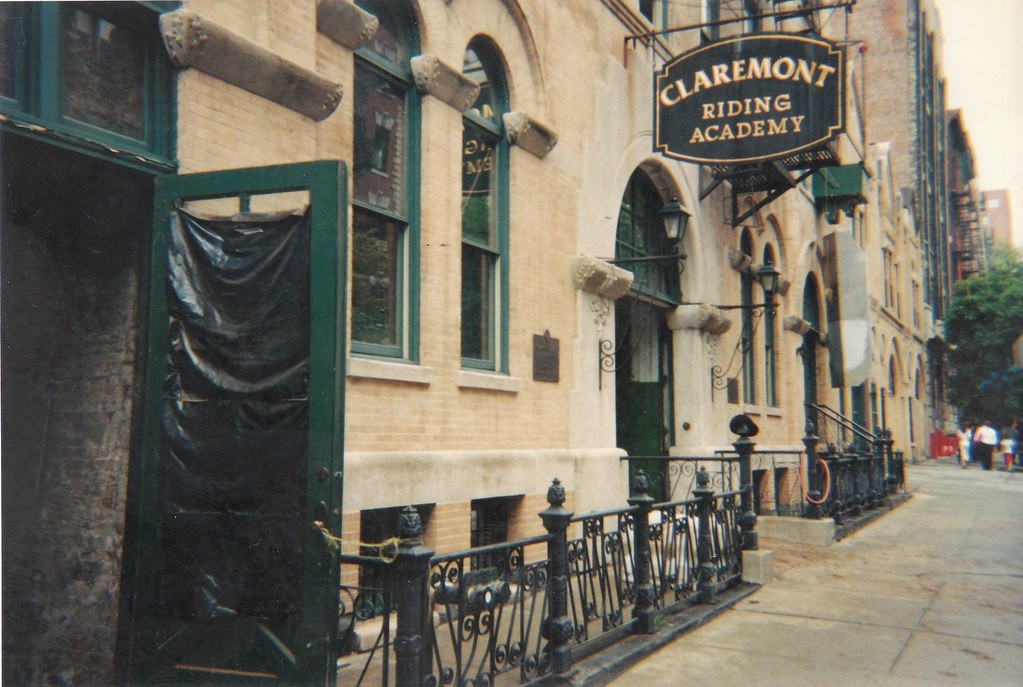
[972,420,998,470]
[999,417,1019,472]
[957,420,976,468]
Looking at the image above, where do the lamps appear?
[607,193,692,273]
[718,261,781,318]
